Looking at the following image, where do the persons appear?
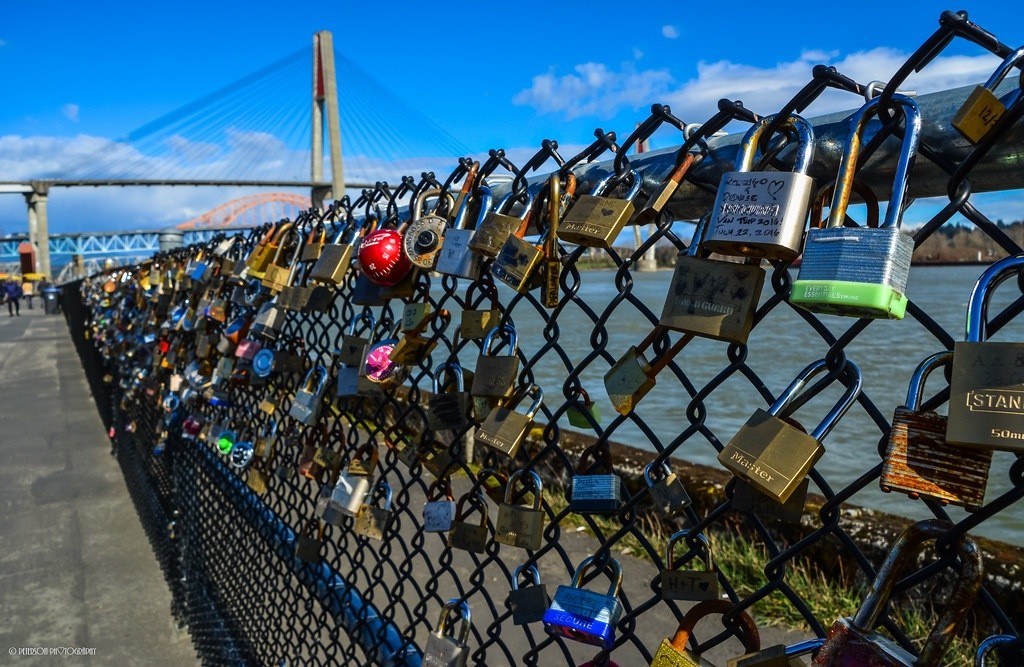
[2,275,21,317]
[36,277,50,309]
[22,279,34,310]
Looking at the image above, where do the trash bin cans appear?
[43,288,62,314]
